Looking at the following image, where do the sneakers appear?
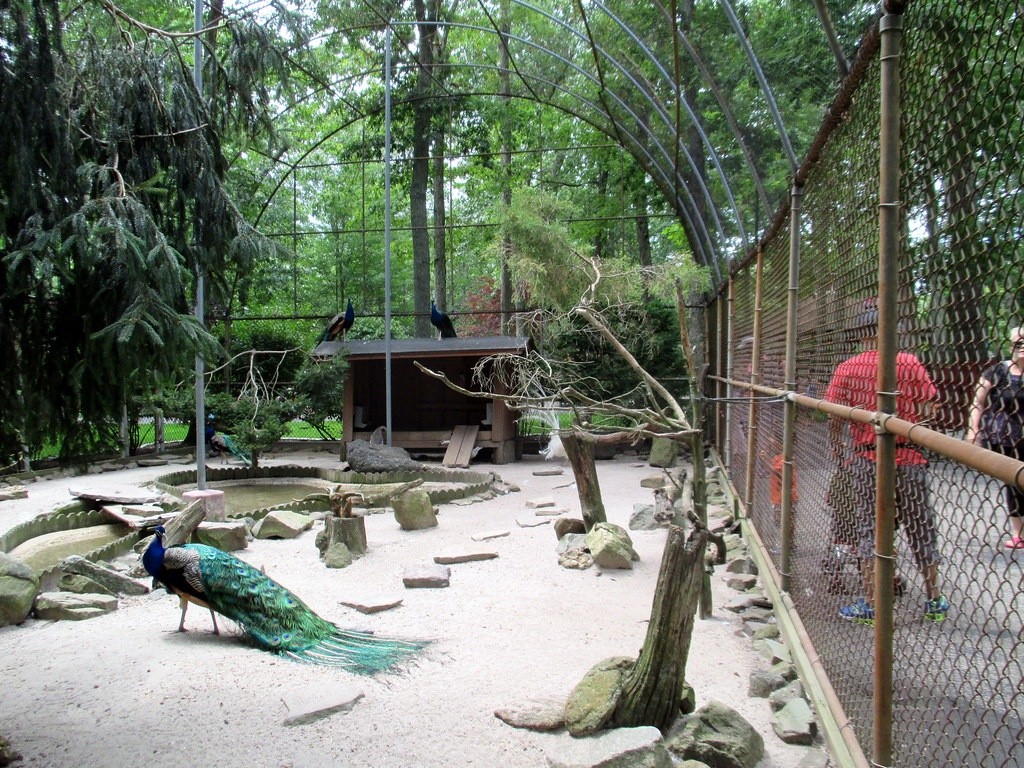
[924,595,948,622]
[839,597,875,627]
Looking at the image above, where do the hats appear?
[850,298,903,329]
[737,337,753,349]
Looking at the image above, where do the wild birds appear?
[430,298,458,337]
[139,516,456,696]
[205,426,252,467]
[314,296,354,350]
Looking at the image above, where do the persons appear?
[732,308,950,624]
[968,327,1024,549]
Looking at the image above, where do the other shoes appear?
[1005,538,1024,549]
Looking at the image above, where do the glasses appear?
[1013,341,1024,348]
